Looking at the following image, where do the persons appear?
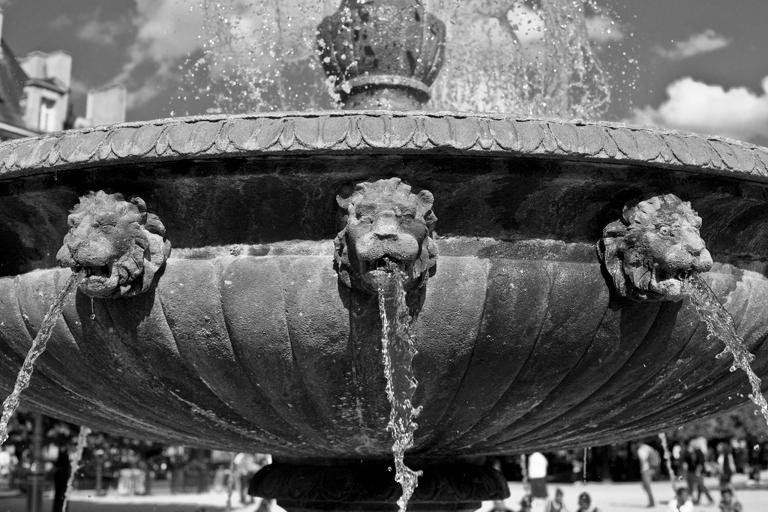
[52,453,71,512]
[487,439,766,511]
[234,453,277,505]
